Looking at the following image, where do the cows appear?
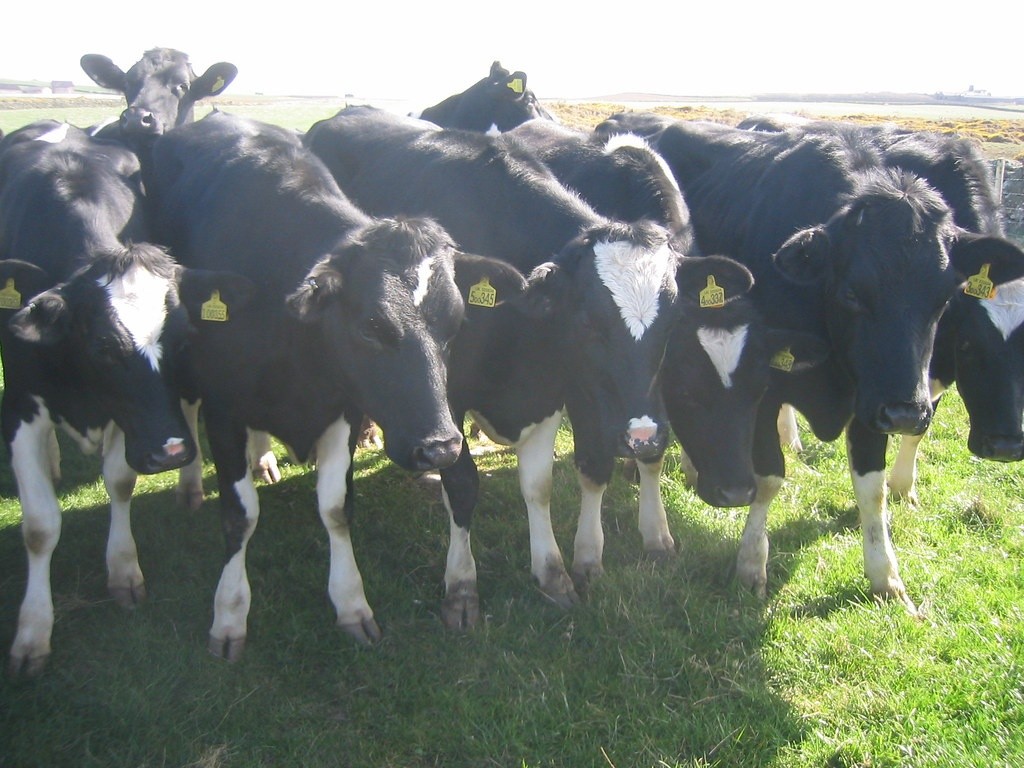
[0,46,1023,685]
